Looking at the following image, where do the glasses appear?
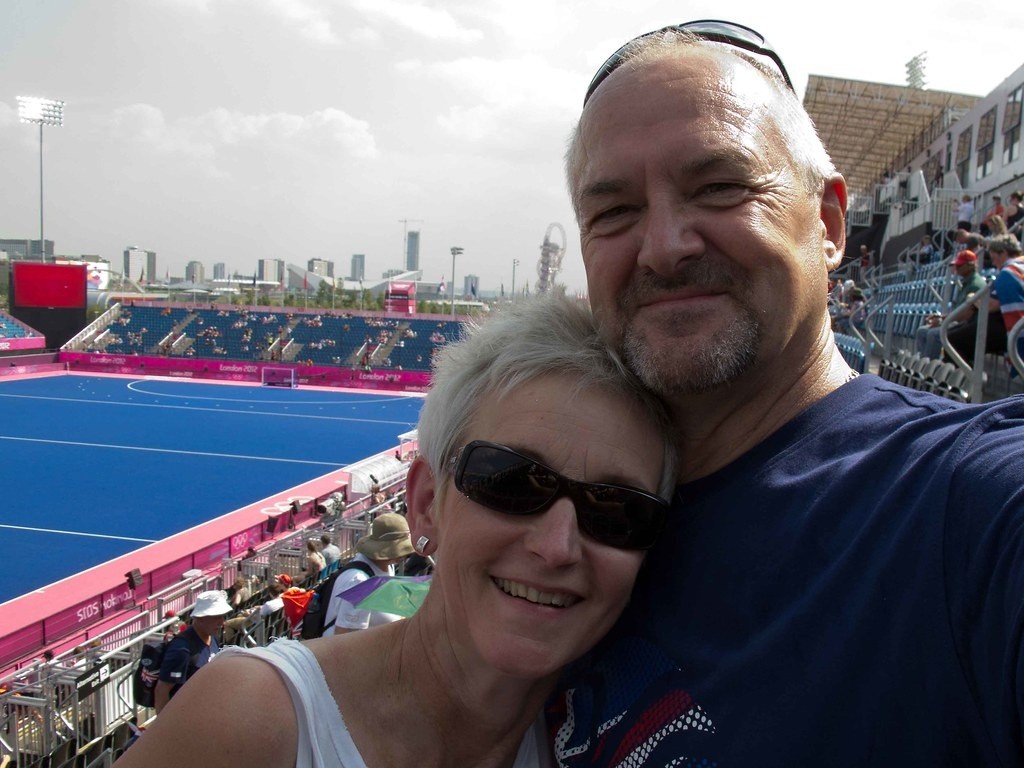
[582,19,797,116]
[452,440,672,552]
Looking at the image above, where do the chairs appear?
[833,257,989,403]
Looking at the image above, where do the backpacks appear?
[301,562,376,640]
[133,638,168,708]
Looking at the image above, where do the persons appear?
[536,20,1024,768]
[112,282,679,767]
[368,482,394,506]
[35,638,102,683]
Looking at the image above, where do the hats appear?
[356,512,418,560]
[274,574,290,585]
[191,590,234,619]
[950,249,976,265]
